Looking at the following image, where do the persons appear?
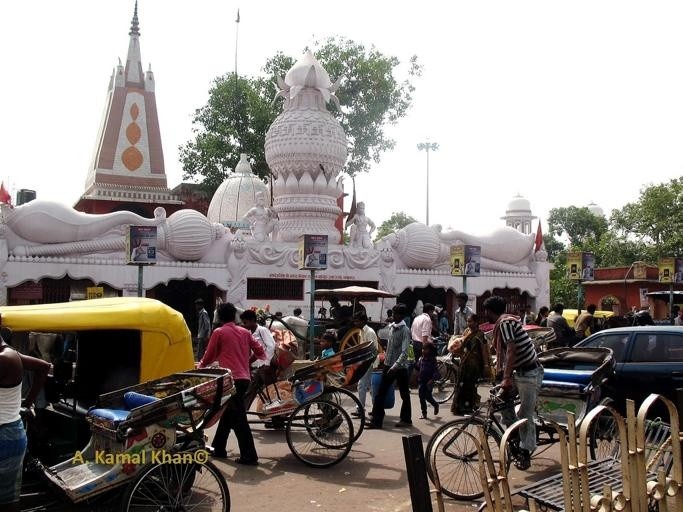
[0,325,51,512]
[484,295,544,470]
[451,315,485,414]
[573,304,598,343]
[345,201,374,248]
[547,304,573,347]
[534,306,550,327]
[417,342,440,419]
[244,189,282,243]
[411,302,447,390]
[523,303,537,324]
[190,294,413,464]
[454,292,475,337]
[438,309,451,337]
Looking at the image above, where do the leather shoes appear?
[434,404,439,414]
[517,453,530,470]
[419,415,426,419]
[351,412,385,429]
[395,421,412,427]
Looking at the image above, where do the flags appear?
[0,182,14,208]
[534,222,542,254]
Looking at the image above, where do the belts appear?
[523,359,540,371]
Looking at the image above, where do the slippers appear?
[212,453,227,458]
[236,458,258,465]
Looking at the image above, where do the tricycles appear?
[1,369,240,512]
[424,336,549,406]
[189,341,384,467]
[426,343,626,498]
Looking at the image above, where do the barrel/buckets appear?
[371,369,394,408]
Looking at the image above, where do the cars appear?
[562,327,681,430]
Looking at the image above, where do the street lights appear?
[416,141,440,224]
